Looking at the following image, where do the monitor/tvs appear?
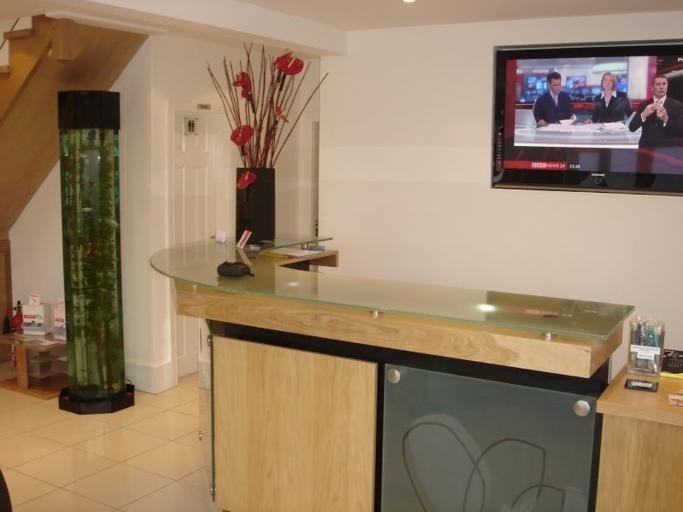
[491,38,683,197]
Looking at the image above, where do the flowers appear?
[208,42,328,190]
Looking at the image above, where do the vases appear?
[235,168,276,244]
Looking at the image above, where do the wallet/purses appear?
[217,261,255,277]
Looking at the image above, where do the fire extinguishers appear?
[3,301,24,367]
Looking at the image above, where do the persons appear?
[584,72,632,124]
[533,71,575,128]
[629,75,683,149]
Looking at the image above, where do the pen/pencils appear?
[630,314,664,373]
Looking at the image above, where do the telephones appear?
[662,349,683,373]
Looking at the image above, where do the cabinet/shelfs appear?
[1,332,70,400]
